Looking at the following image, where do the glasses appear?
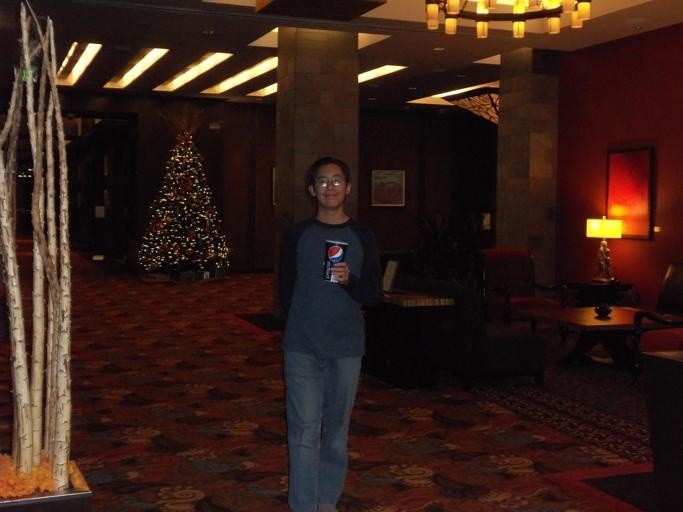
[314,176,345,188]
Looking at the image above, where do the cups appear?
[321,238,349,285]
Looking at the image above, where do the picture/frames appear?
[605,146,657,242]
[370,169,407,208]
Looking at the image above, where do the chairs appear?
[630,261,683,386]
[475,247,569,348]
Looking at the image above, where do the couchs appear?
[392,277,545,392]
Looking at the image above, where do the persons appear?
[275,155,386,512]
